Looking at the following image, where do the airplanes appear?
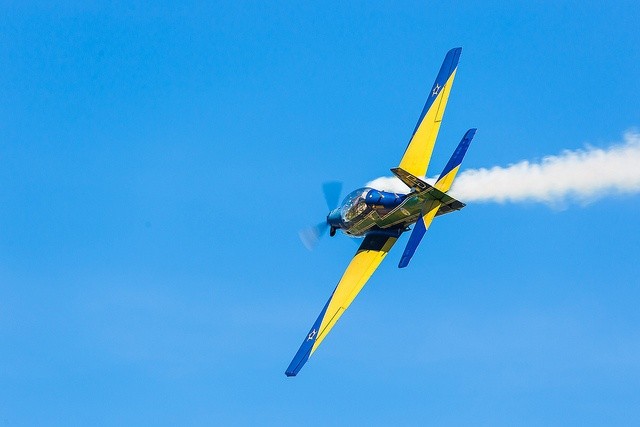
[284,46,477,378]
